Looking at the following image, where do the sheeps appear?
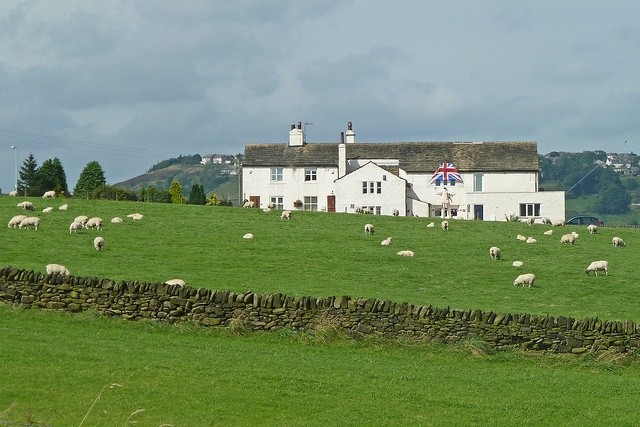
[10,191,18,197]
[75,216,88,228]
[586,260,609,277]
[267,202,274,208]
[441,220,449,231]
[16,201,33,210]
[392,208,399,218]
[545,221,550,224]
[561,232,574,249]
[127,213,144,220]
[544,230,553,235]
[516,235,526,240]
[19,216,41,230]
[85,217,103,231]
[42,190,56,199]
[243,201,254,208]
[165,279,186,288]
[42,206,53,212]
[110,216,123,223]
[69,221,82,234]
[243,233,253,239]
[527,218,535,226]
[93,236,105,251]
[380,237,393,246]
[396,250,414,257]
[427,222,435,228]
[513,274,535,288]
[587,225,597,234]
[8,215,26,229]
[365,223,374,234]
[280,210,292,220]
[571,232,582,239]
[263,208,271,212]
[489,247,501,260]
[46,263,70,276]
[513,261,523,266]
[526,237,536,244]
[612,237,627,248]
[58,204,68,211]
